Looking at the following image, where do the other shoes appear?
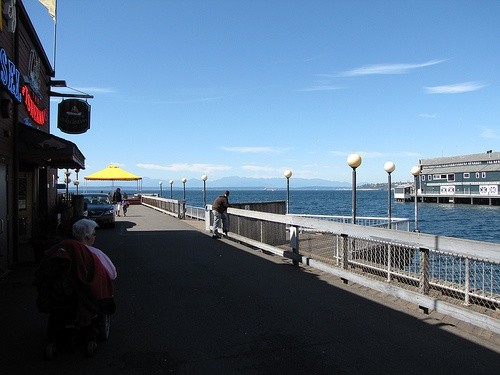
[212,235,221,238]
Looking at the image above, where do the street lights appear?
[410,166,422,230]
[159,181,163,197]
[169,180,173,199]
[201,175,208,208]
[384,161,396,229]
[181,178,187,200]
[347,154,362,226]
[284,170,293,214]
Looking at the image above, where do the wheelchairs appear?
[37,242,110,358]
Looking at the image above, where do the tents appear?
[84,166,142,196]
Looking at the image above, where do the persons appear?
[122,200,129,216]
[210,190,230,239]
[73,218,117,280]
[112,188,122,217]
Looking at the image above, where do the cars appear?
[77,192,116,227]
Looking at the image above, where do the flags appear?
[38,0,57,22]
